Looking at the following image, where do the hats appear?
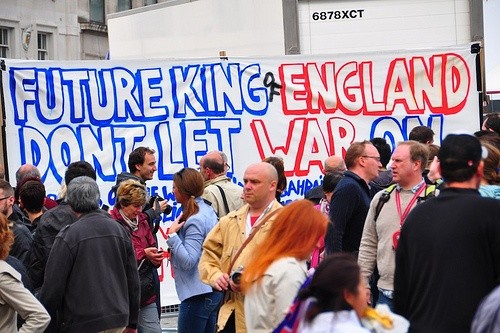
[438,134,482,167]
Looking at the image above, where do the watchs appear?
[167,233,177,240]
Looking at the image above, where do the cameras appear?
[227,266,244,292]
[150,195,172,215]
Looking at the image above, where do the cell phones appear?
[157,250,164,254]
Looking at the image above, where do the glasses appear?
[362,155,380,161]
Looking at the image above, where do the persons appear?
[393,134,500,333]
[23,161,112,294]
[408,112,500,199]
[1,160,111,227]
[0,181,36,270]
[218,151,228,175]
[199,162,286,333]
[0,210,51,333]
[324,140,442,302]
[38,176,140,333]
[108,179,166,333]
[112,145,171,323]
[295,252,410,333]
[261,157,284,201]
[237,199,330,333]
[166,167,227,333]
[356,139,441,312]
[366,137,395,198]
[195,151,245,219]
[308,172,343,270]
[304,155,347,206]
